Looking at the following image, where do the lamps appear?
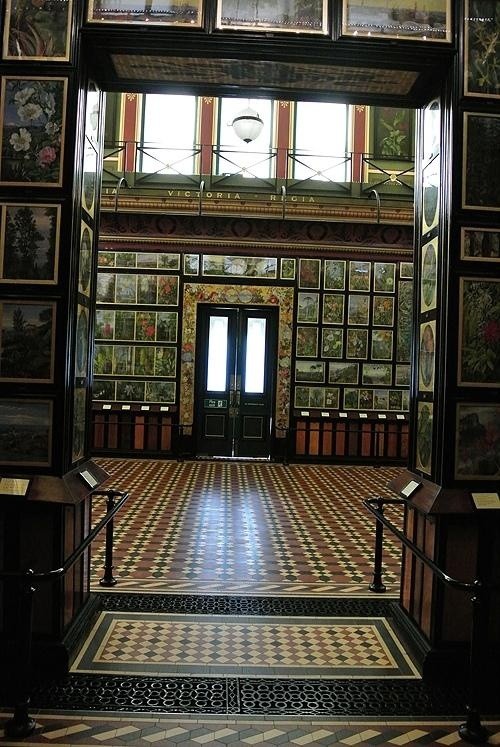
[228,98,265,144]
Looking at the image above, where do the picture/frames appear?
[293,258,413,410]
[90,250,181,404]
[184,253,296,280]
[104,92,119,149]
[336,0,457,45]
[210,0,334,40]
[374,107,412,161]
[86,0,206,28]
